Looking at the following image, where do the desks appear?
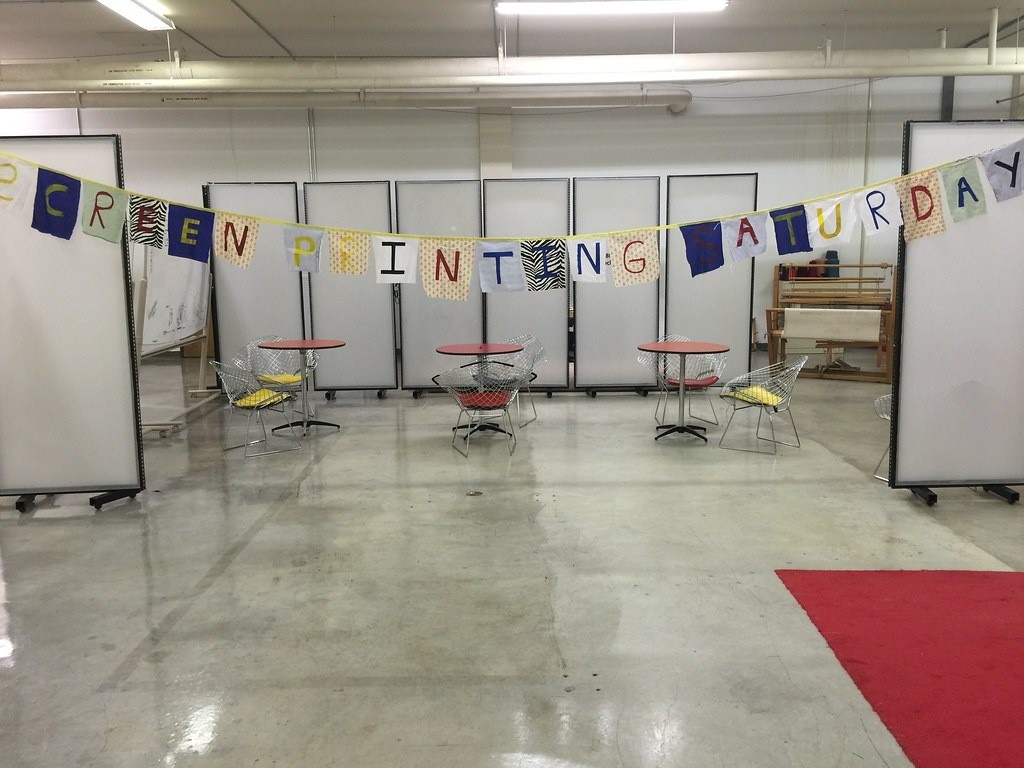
[637,342,731,442]
[259,340,346,437]
[436,344,525,440]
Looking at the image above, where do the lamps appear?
[98,0,176,31]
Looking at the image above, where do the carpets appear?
[774,569,1024,768]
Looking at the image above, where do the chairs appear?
[717,354,809,455]
[637,333,727,426]
[208,360,303,458]
[873,393,892,482]
[468,334,548,429]
[232,335,321,424]
[432,359,533,457]
